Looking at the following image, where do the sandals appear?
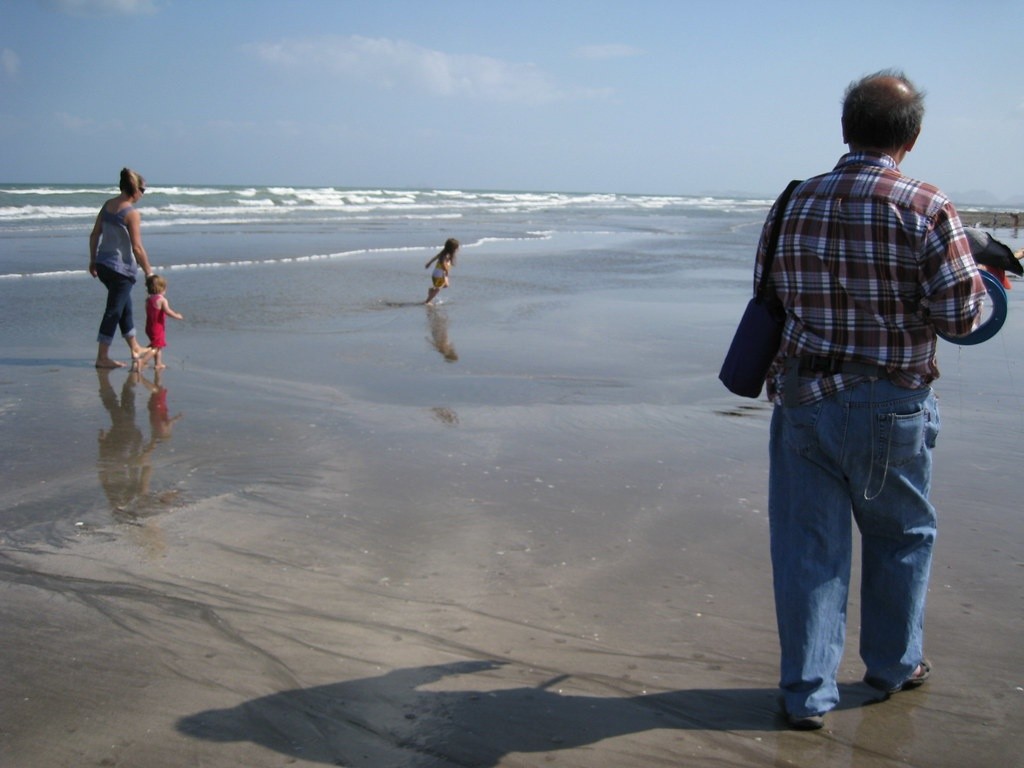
[779,693,825,731]
[862,659,932,693]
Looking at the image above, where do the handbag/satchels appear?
[718,178,801,398]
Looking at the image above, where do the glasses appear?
[138,187,145,194]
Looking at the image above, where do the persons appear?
[137,274,183,370]
[424,305,460,363]
[992,214,997,230]
[131,370,185,440]
[1010,213,1019,227]
[750,66,1004,732]
[96,367,158,509]
[89,166,152,366]
[424,237,460,307]
